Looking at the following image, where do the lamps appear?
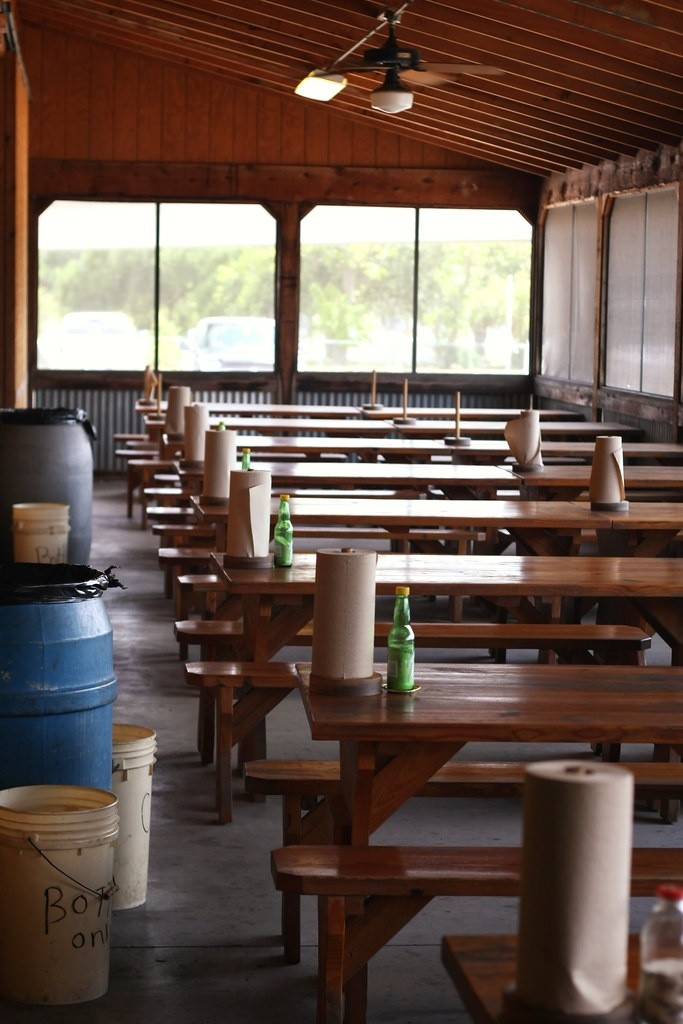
[361,8,420,114]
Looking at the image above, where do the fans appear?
[306,10,505,86]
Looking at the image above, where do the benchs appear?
[116,431,683,1024]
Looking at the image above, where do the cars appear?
[178,315,286,371]
[36,309,152,369]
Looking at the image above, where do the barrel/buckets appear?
[12,503,71,563]
[112,724,156,911]
[0,786,120,1004]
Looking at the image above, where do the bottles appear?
[274,495,293,566]
[631,884,683,1024]
[243,449,250,469]
[388,587,415,691]
[219,422,226,431]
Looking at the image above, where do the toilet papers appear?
[183,405,210,461]
[588,435,627,504]
[504,410,543,466]
[227,469,274,558]
[202,428,239,497]
[311,545,380,680]
[516,757,631,1022]
[164,385,192,435]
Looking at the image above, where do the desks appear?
[438,437,683,553]
[193,494,613,559]
[143,414,396,460]
[357,404,587,463]
[174,457,524,614]
[439,933,683,1024]
[575,500,683,558]
[160,432,452,464]
[388,416,645,497]
[135,398,365,420]
[293,656,683,1024]
[209,546,683,803]
[500,464,683,645]
